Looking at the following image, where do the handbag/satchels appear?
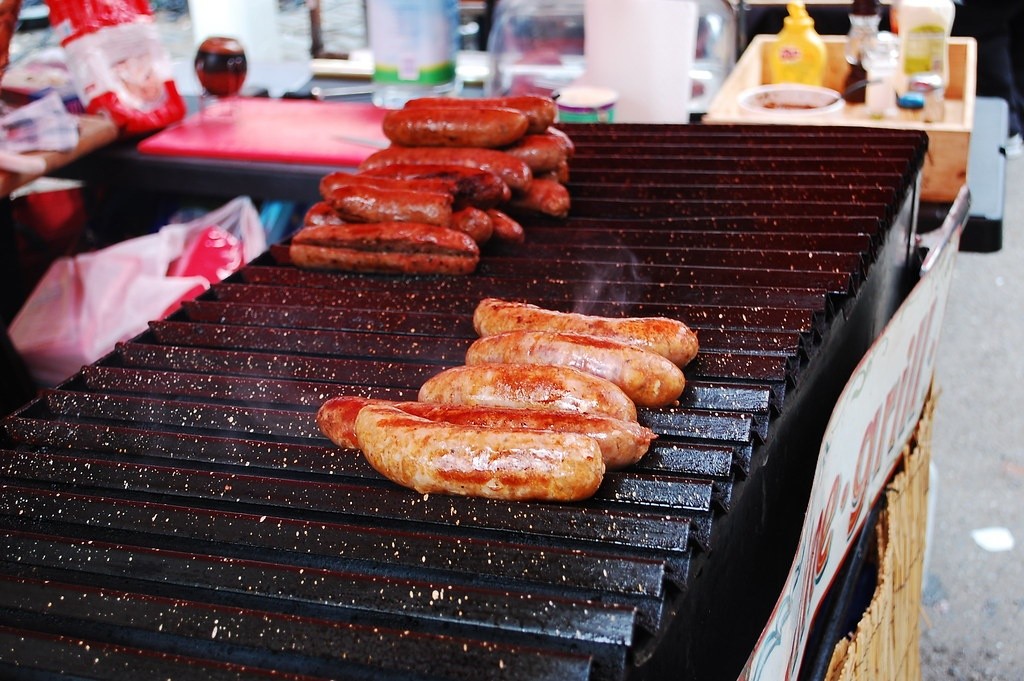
[42,0,189,142]
[7,196,268,387]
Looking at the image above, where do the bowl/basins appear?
[738,83,846,115]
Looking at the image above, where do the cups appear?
[189,0,279,97]
[550,86,617,122]
[581,0,700,124]
[364,0,457,110]
[459,0,487,50]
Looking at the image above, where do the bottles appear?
[768,0,827,86]
[7,6,51,102]
[896,92,925,122]
[842,0,883,104]
[459,81,485,99]
[911,73,945,123]
[865,30,896,110]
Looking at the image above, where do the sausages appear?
[318,298,698,502]
[289,95,574,277]
